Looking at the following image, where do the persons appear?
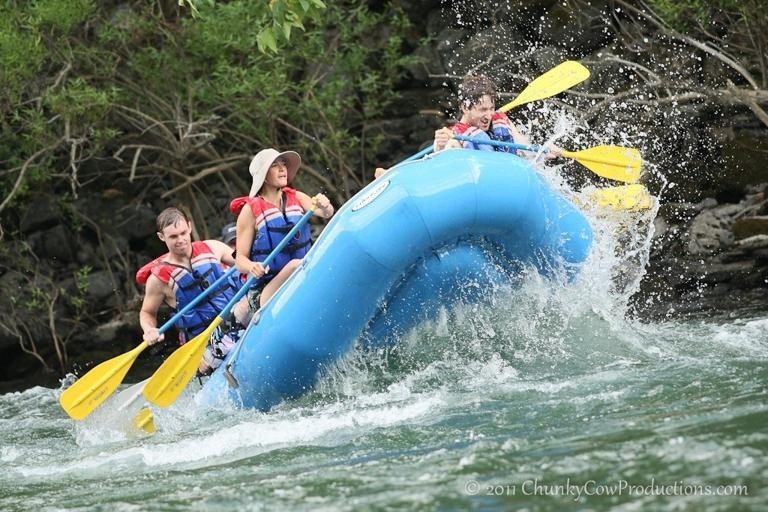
[220,220,239,250]
[236,148,333,312]
[139,207,237,377]
[434,75,557,163]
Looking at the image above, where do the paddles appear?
[379,60,589,172]
[58,264,238,420]
[440,128,642,183]
[131,407,157,433]
[144,194,325,407]
[570,184,652,214]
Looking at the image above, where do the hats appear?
[248,149,302,199]
[219,222,237,243]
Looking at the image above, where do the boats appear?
[186,149,594,414]
